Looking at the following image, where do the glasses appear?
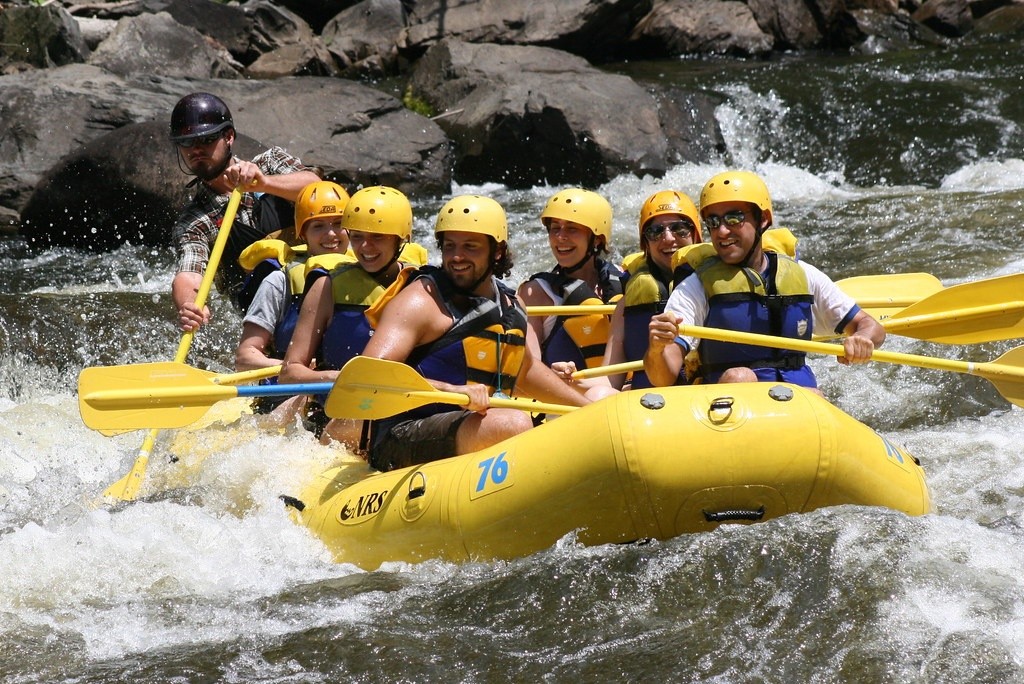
[177,129,225,147]
[641,221,696,242]
[705,210,756,229]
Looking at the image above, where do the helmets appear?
[435,194,508,245]
[541,188,613,254]
[169,92,237,142]
[342,185,412,244]
[639,190,702,244]
[701,172,773,227]
[295,181,350,240]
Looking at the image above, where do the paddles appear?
[98,365,280,439]
[322,355,581,430]
[570,273,1023,381]
[678,321,1024,408]
[99,185,248,500]
[524,273,945,321]
[77,361,331,432]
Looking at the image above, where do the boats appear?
[164,382,938,574]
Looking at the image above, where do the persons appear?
[168,92,323,332]
[235,181,427,438]
[603,169,886,392]
[517,191,633,421]
[320,195,595,473]
